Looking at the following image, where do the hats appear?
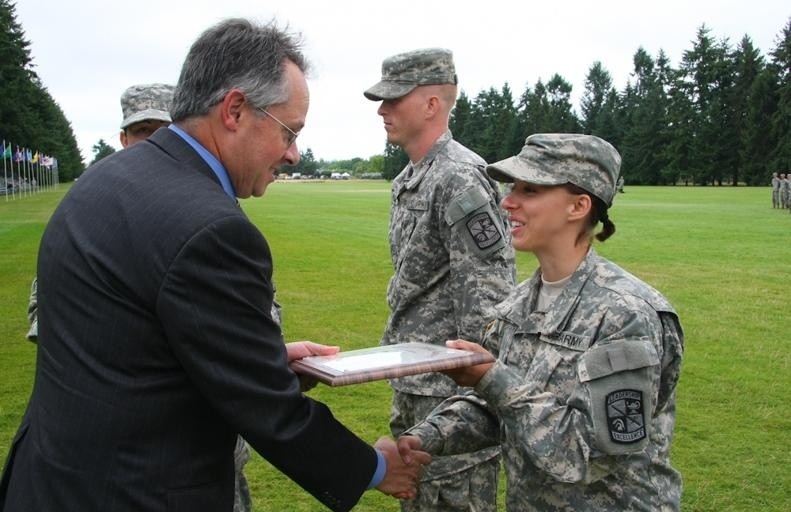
[120,82,175,130]
[364,47,457,102]
[485,133,623,208]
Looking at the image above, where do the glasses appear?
[226,87,300,150]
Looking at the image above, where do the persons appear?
[771,171,781,209]
[779,173,787,208]
[24,83,179,346]
[394,132,687,511]
[361,48,518,511]
[786,174,791,208]
[0,12,433,511]
[619,176,625,193]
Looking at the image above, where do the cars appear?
[276,172,382,180]
[0,172,30,195]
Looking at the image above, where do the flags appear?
[1,140,59,170]
